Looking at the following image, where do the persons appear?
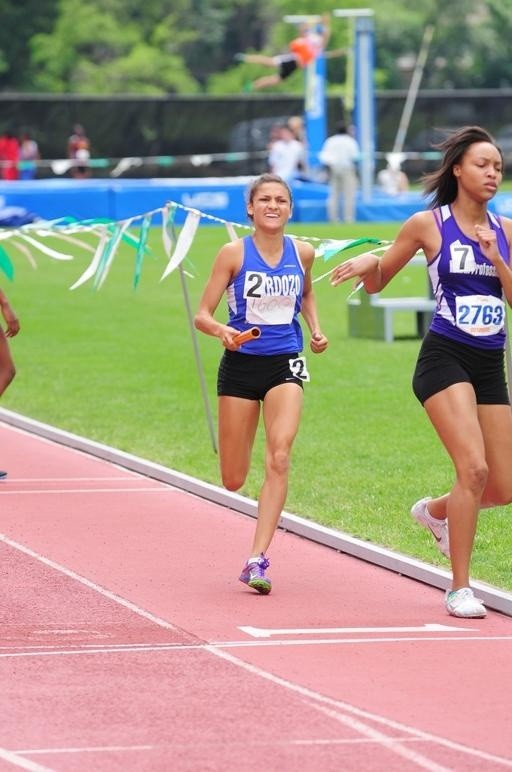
[320,124,364,225]
[0,289,23,481]
[231,10,334,98]
[67,122,92,178]
[194,171,329,596]
[17,129,39,179]
[265,117,309,184]
[375,161,409,192]
[1,131,20,180]
[327,125,512,619]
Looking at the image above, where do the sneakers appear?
[444,582,487,619]
[238,552,273,595]
[410,495,451,560]
